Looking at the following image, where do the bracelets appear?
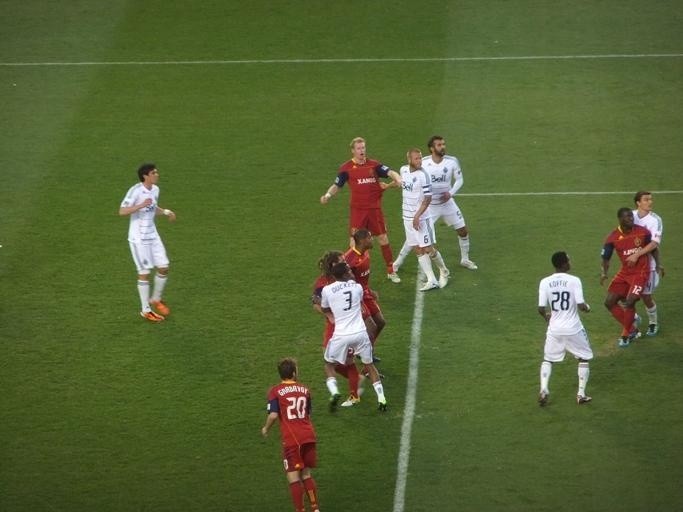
[164,208,169,215]
[325,192,332,199]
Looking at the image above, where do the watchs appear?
[116,162,176,322]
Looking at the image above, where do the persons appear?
[599,205,665,348]
[618,193,666,337]
[536,251,594,406]
[260,358,322,511]
[385,135,479,281]
[308,224,389,413]
[320,136,406,283]
[376,149,451,293]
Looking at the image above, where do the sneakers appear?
[341,395,360,407]
[646,323,660,337]
[361,370,384,379]
[329,391,341,411]
[356,353,381,363]
[139,311,164,322]
[538,389,550,407]
[629,328,639,341]
[419,281,439,291]
[150,299,169,315]
[633,316,642,328]
[617,335,630,346]
[438,266,450,289]
[387,273,400,284]
[377,400,387,413]
[460,259,479,270]
[576,392,591,405]
